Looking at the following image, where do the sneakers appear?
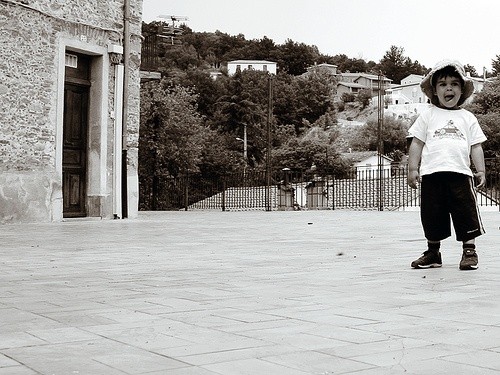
[411,249,443,268]
[459,248,478,270]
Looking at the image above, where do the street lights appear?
[236,121,248,176]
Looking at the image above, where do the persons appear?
[406,58,487,269]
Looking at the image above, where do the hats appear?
[419,58,475,106]
